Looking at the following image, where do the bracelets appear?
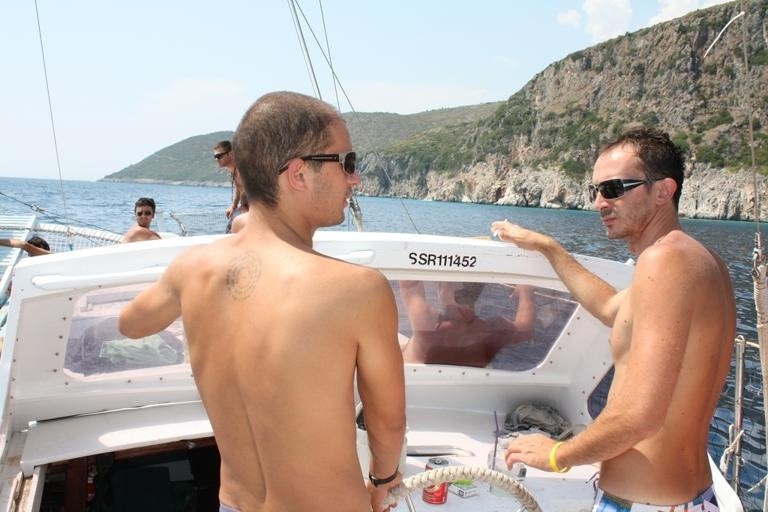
[548,442,573,474]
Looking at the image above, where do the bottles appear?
[87,470,97,511]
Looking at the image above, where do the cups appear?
[488,451,519,497]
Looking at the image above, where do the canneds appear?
[422,457,449,504]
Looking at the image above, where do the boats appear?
[0,214,767,512]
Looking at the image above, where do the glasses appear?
[276,151,356,176]
[589,177,648,203]
[214,152,227,159]
[137,210,151,216]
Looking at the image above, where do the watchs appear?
[367,464,401,488]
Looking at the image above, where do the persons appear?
[488,127,737,512]
[119,197,162,244]
[212,140,249,235]
[395,279,539,368]
[119,91,407,512]
[0,237,54,358]
